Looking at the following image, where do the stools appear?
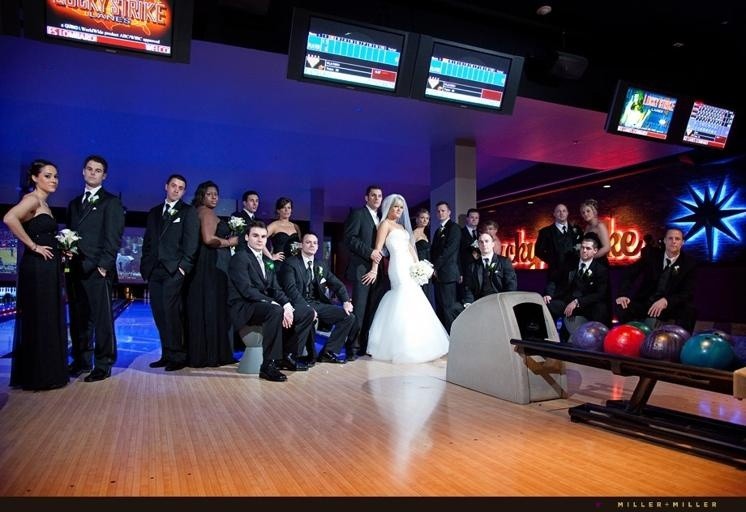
[234,325,263,374]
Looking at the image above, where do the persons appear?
[64,157,125,382]
[535,204,583,272]
[2,159,73,391]
[615,228,695,335]
[136,172,519,382]
[543,232,610,333]
[579,199,611,259]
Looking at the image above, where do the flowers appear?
[574,243,582,250]
[410,260,434,286]
[289,241,302,256]
[168,207,179,216]
[266,260,275,273]
[489,263,498,273]
[318,266,324,276]
[227,215,246,249]
[585,269,592,277]
[88,194,99,204]
[471,239,479,248]
[55,229,83,273]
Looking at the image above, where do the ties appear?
[562,225,567,235]
[162,204,171,224]
[257,253,266,279]
[306,260,312,296]
[578,262,586,276]
[252,215,256,221]
[483,257,490,270]
[472,230,477,240]
[80,191,91,210]
[663,257,672,273]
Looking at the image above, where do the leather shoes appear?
[258,364,288,382]
[149,358,166,368]
[69,362,93,377]
[165,362,187,371]
[306,358,317,367]
[274,357,309,371]
[318,350,345,363]
[83,367,112,383]
[346,348,359,361]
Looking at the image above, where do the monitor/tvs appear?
[410,32,526,118]
[284,12,411,106]
[603,79,682,147]
[677,96,738,155]
[27,0,192,67]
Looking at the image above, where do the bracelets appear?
[32,244,37,251]
